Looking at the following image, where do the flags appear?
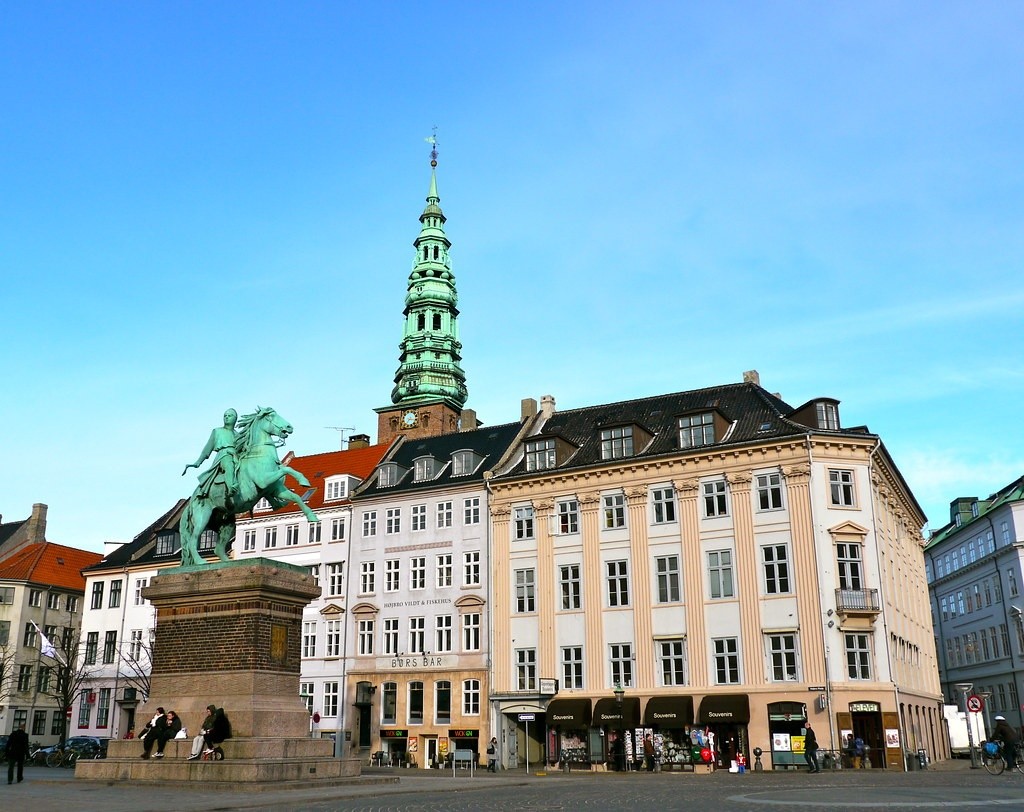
[30,619,56,658]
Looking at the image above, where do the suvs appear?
[40,735,115,753]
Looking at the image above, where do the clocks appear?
[401,410,418,428]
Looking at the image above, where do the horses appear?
[178,407,320,565]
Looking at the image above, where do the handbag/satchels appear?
[1,752,8,762]
[983,742,998,755]
[487,742,494,754]
[138,726,151,740]
[174,728,187,739]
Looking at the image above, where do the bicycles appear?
[45,744,107,768]
[981,740,1024,775]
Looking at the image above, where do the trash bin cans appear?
[908,753,920,771]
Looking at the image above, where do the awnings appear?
[593,697,640,730]
[699,694,750,724]
[546,699,591,730]
[645,696,694,730]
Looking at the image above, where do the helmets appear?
[994,715,1005,721]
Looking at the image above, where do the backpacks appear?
[214,745,224,760]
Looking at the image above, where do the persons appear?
[193,408,290,512]
[986,716,1018,772]
[644,734,656,772]
[737,750,746,773]
[841,734,856,764]
[4,723,30,784]
[804,722,821,774]
[138,707,181,760]
[187,705,229,761]
[486,737,499,773]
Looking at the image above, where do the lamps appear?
[827,609,833,616]
[828,620,835,628]
[632,653,636,660]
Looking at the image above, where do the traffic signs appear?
[518,714,535,721]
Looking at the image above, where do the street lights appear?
[978,691,992,736]
[955,683,978,769]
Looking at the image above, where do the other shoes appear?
[141,751,150,760]
[151,751,165,757]
[1005,767,1013,771]
[487,768,489,772]
[492,771,496,773]
[187,753,198,760]
[202,746,214,755]
[809,768,821,773]
